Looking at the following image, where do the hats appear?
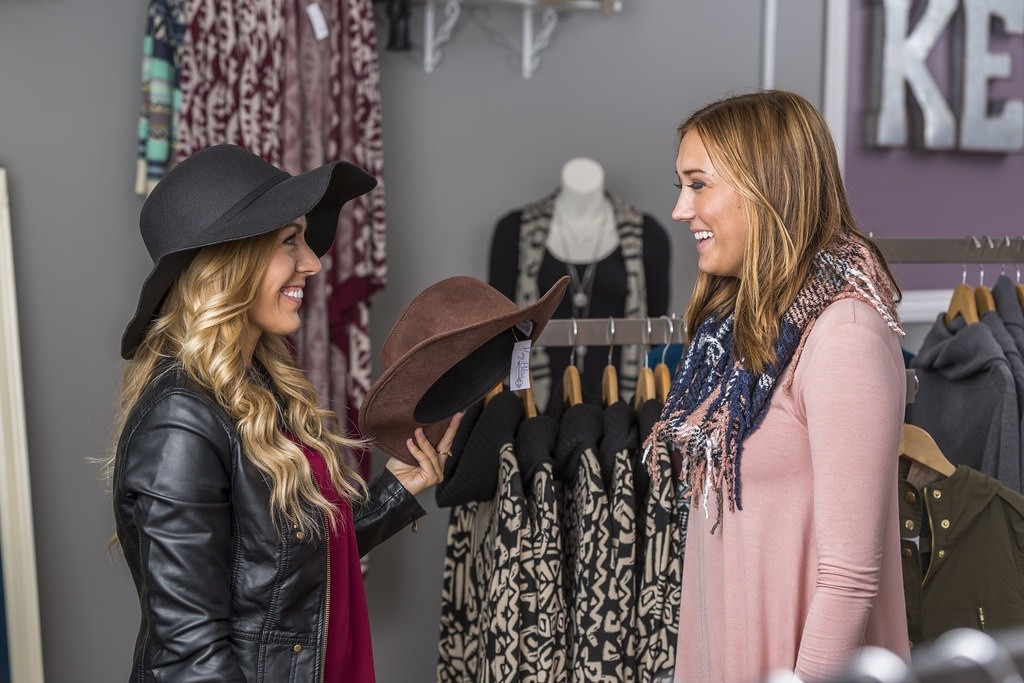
[121,144,377,359]
[356,275,569,466]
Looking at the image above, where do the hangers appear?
[484,314,689,420]
[899,377,957,477]
[943,234,1024,327]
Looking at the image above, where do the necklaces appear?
[555,200,610,373]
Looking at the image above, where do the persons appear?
[488,157,672,408]
[113,143,464,683]
[643,88,910,683]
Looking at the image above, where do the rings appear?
[437,451,452,456]
